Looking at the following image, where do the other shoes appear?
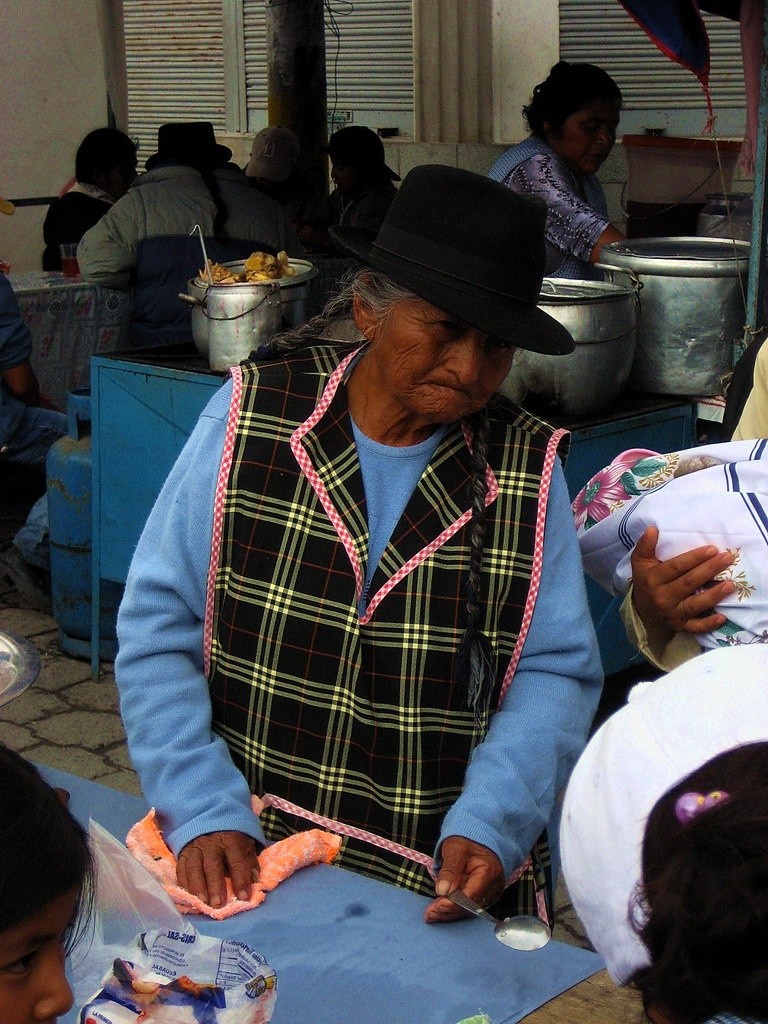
[1,545,51,612]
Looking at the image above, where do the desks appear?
[35,762,650,1024]
[6,270,135,413]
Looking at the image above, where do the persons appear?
[114,164,606,920]
[243,124,310,222]
[328,126,403,257]
[43,127,139,273]
[0,268,68,615]
[559,643,768,1024]
[619,331,768,672]
[76,122,301,349]
[0,744,97,1024]
[487,61,628,280]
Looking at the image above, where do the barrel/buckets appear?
[202,281,284,373]
[595,237,752,396]
[696,193,755,242]
[621,134,743,220]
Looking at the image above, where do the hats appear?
[331,126,400,182]
[245,125,299,181]
[144,122,232,172]
[327,163,575,356]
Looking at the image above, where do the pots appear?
[498,273,637,418]
[177,259,320,361]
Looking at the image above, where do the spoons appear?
[448,889,551,951]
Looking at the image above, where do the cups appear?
[60,243,80,278]
